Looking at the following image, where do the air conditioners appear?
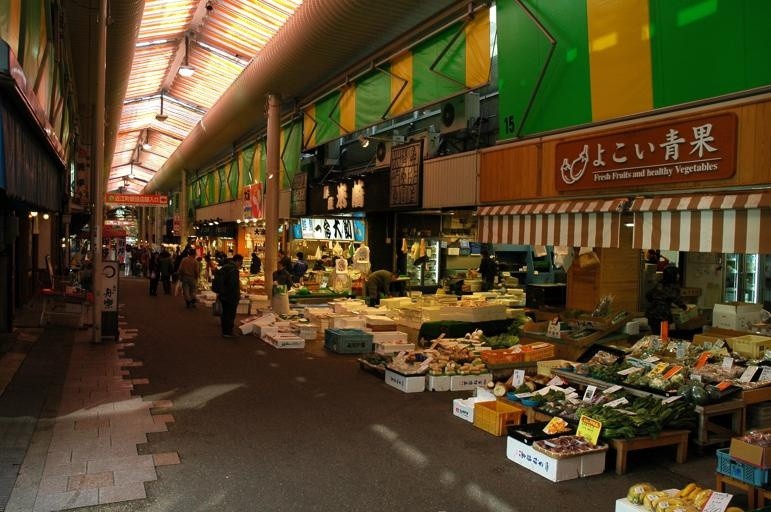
[440,93,481,134]
[372,140,391,168]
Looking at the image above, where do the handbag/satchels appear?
[212,300,223,316]
[211,269,221,293]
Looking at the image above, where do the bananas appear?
[628,483,743,512]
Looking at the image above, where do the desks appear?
[38,287,95,330]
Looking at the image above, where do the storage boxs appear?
[238,286,531,353]
[711,298,764,333]
[614,426,771,512]
[452,335,771,484]
[374,335,557,393]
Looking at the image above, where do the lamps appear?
[358,134,412,148]
[192,218,223,230]
[118,29,197,194]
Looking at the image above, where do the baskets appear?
[480,341,555,364]
[716,448,770,488]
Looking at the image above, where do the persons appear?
[656,250,671,272]
[367,268,400,306]
[124,242,261,338]
[291,251,309,282]
[310,259,326,278]
[274,260,293,294]
[279,249,294,273]
[322,254,331,266]
[645,249,659,264]
[645,266,698,335]
[330,256,339,267]
